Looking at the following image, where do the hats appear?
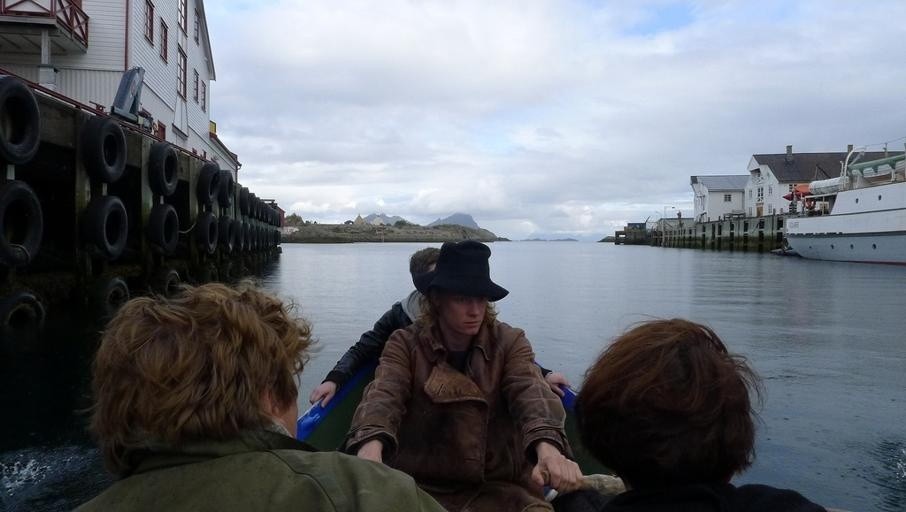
[415,241,509,301]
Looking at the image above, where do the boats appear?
[785,145,905,264]
[288,353,614,474]
[769,244,796,254]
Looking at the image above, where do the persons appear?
[310,247,571,408]
[345,240,584,512]
[71,284,449,512]
[576,319,827,512]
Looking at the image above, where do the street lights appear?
[663,205,675,218]
